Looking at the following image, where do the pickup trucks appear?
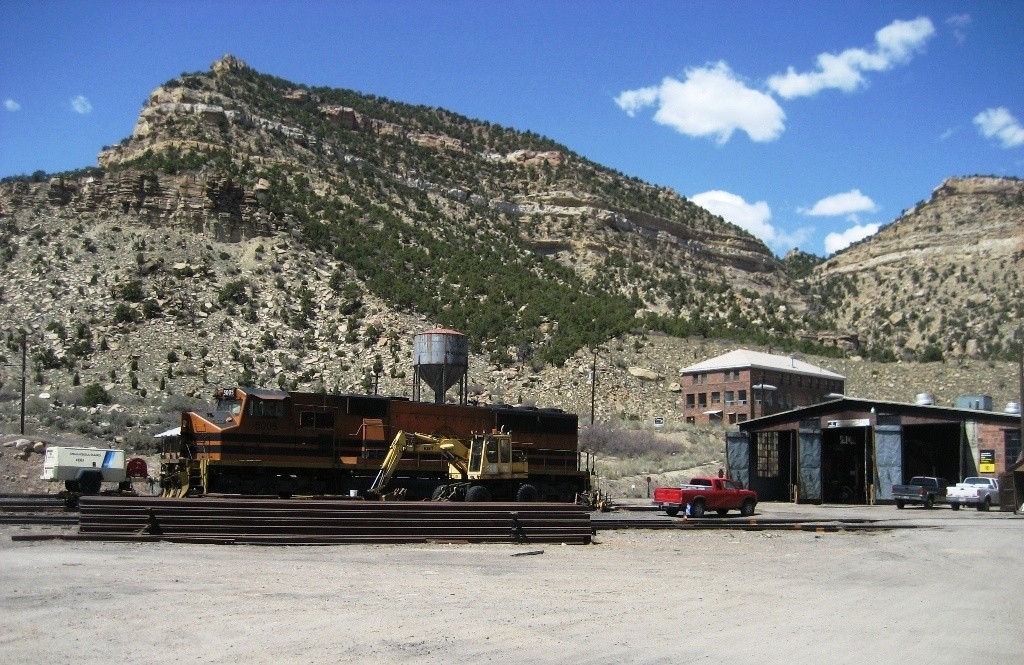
[946,476,1002,511]
[652,476,765,519]
[891,476,955,505]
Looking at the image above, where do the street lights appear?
[591,344,599,425]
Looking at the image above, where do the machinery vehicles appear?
[358,426,545,502]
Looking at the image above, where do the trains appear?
[169,384,592,501]
